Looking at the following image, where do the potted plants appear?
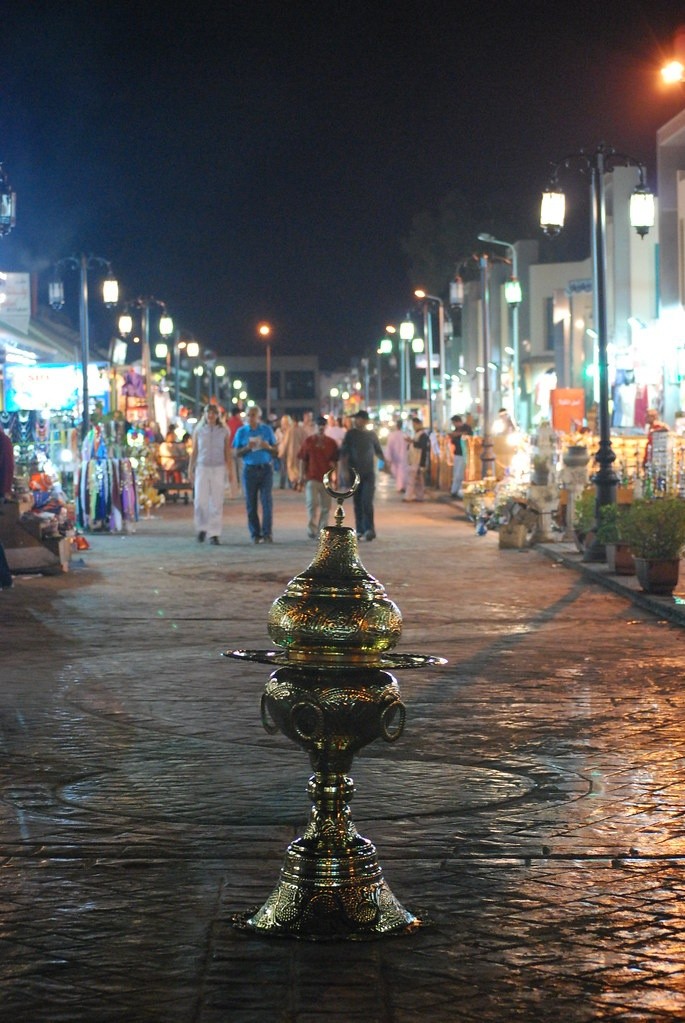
[631,494,685,596]
[597,502,636,576]
[570,484,597,553]
[530,453,550,485]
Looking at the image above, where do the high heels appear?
[356,531,363,538]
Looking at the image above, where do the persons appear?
[159,430,193,499]
[0,429,15,591]
[187,402,431,544]
[642,409,670,469]
[447,408,516,499]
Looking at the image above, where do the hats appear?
[351,410,369,420]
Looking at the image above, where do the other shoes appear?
[210,536,219,545]
[365,530,375,540]
[308,531,315,538]
[263,533,273,544]
[198,530,206,541]
[251,535,260,544]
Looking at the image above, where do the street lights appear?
[189,349,255,423]
[48,249,120,443]
[259,325,273,422]
[539,141,656,561]
[329,289,445,436]
[154,325,200,422]
[478,233,520,425]
[118,290,174,423]
